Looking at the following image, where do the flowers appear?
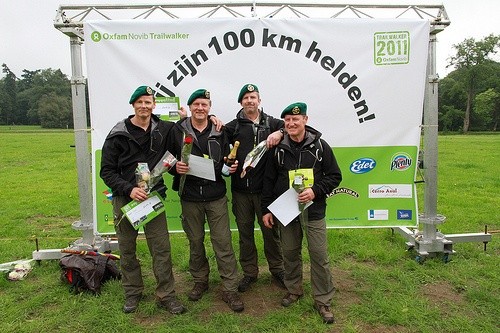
[179,137,192,198]
[115,162,171,227]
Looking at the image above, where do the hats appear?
[129,85,154,104]
[281,102,307,119]
[187,89,210,105]
[238,84,259,103]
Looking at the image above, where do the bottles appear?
[221,140,240,177]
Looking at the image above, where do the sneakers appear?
[222,291,244,312]
[188,283,209,301]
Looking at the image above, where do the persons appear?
[176,84,288,293]
[262,102,342,324]
[167,88,245,312]
[99,85,222,315]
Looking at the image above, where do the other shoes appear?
[313,300,334,324]
[271,272,287,290]
[237,276,258,292]
[155,295,184,314]
[281,292,304,307]
[123,294,143,313]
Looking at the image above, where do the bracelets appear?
[280,128,284,133]
